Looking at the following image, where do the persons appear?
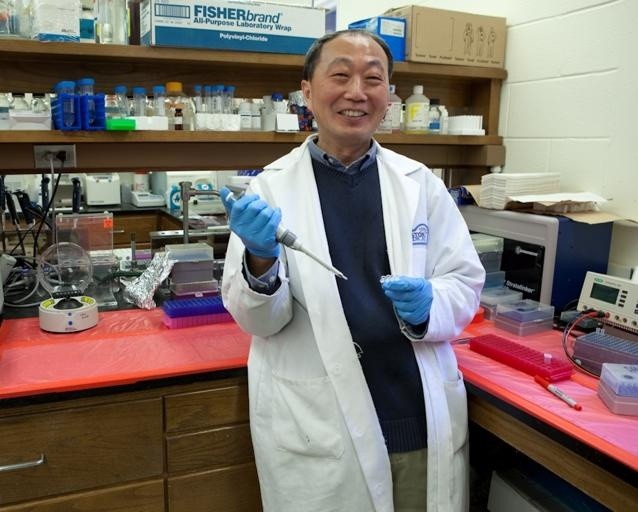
[221,30,485,511]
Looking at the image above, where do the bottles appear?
[53,78,449,135]
[99,23,113,44]
[79,7,96,44]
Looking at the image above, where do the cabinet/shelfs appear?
[0,39,638,512]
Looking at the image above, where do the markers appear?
[533,375,583,412]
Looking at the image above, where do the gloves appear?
[383,276,433,325]
[220,187,282,259]
